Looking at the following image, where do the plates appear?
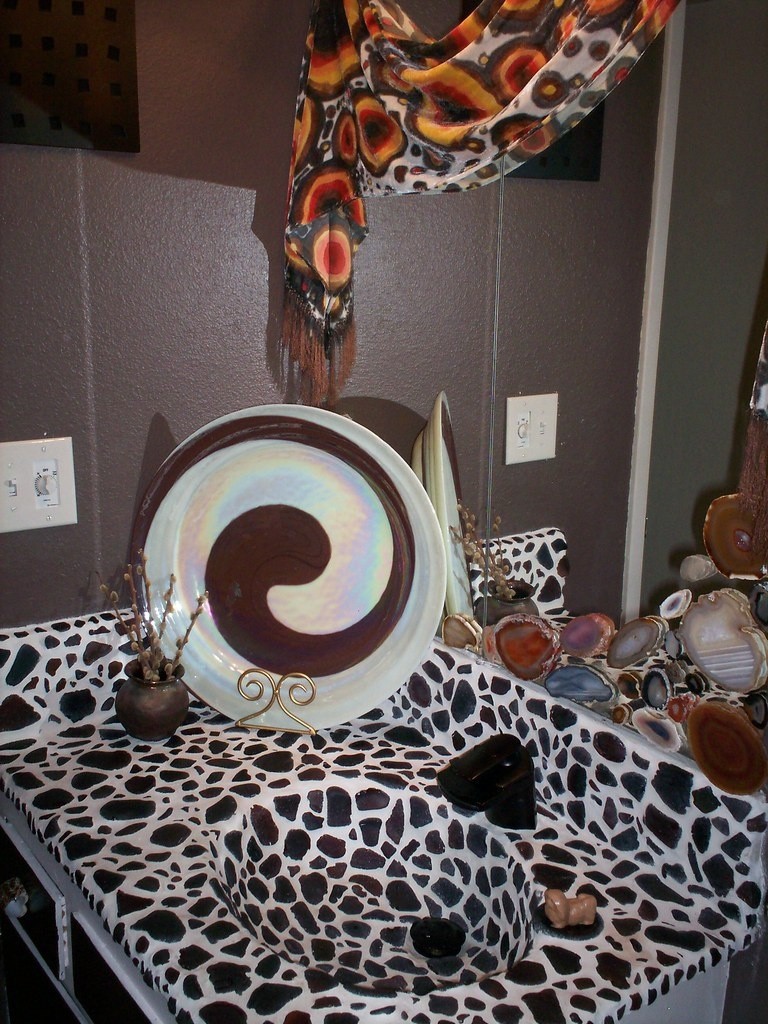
[130,403,447,732]
[409,389,474,621]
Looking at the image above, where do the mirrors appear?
[481,0,768,797]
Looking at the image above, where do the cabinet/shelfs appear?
[0,790,732,1024]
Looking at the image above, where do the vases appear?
[115,656,189,740]
